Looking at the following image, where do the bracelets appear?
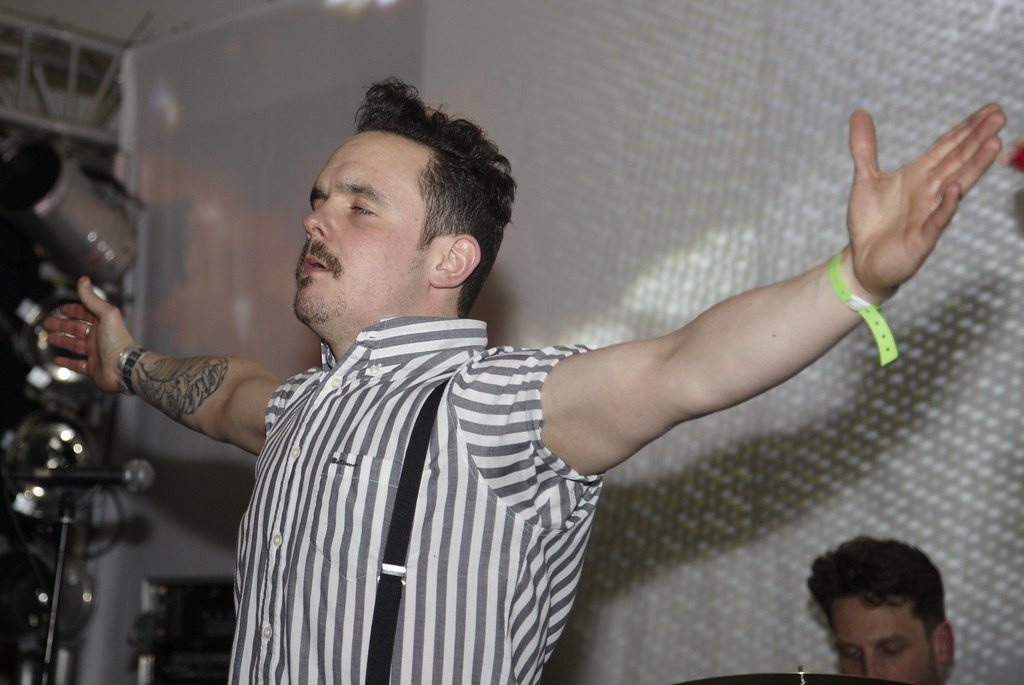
[118,345,145,396]
[830,250,899,365]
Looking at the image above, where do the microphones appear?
[11,459,155,493]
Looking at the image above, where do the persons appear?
[45,73,1006,685]
[804,536,955,685]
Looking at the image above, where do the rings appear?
[82,361,89,375]
[83,324,91,337]
